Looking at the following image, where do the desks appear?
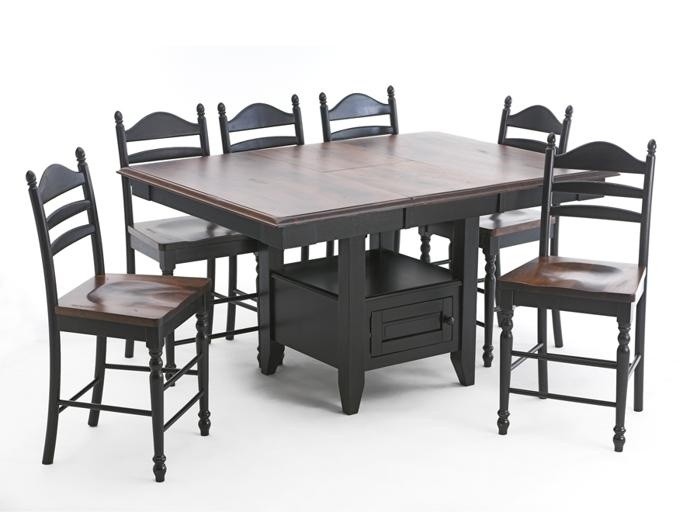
[118,132,620,414]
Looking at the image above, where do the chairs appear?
[114,103,260,386]
[420,96,572,368]
[216,93,309,342]
[318,85,399,257]
[497,134,656,451]
[26,147,214,483]
